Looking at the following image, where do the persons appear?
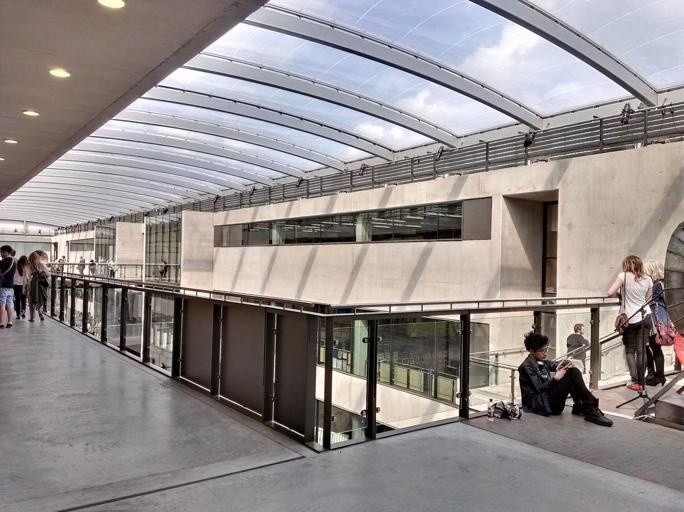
[643,258,668,387]
[0,245,48,328]
[157,259,165,281]
[566,323,589,374]
[517,332,613,426]
[607,255,654,390]
[54,255,116,278]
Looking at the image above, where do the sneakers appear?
[0,314,44,329]
[627,383,643,392]
[572,404,613,425]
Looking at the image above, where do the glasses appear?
[538,347,549,352]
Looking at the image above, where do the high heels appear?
[645,372,666,386]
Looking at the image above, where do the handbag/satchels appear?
[615,314,629,333]
[654,320,676,346]
